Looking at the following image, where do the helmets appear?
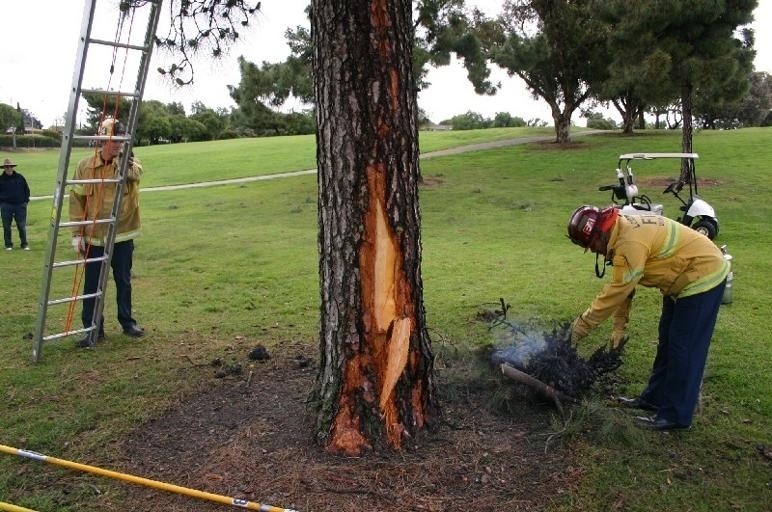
[98,118,126,140]
[564,205,605,255]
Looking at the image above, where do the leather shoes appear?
[635,412,691,432]
[616,393,666,411]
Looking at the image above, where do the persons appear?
[69,119,144,348]
[564,203,729,430]
[1,158,32,251]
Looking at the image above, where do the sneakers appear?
[123,325,144,338]
[75,332,105,347]
[5,246,32,251]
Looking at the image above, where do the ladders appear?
[31,0,162,368]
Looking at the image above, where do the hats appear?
[0,158,18,169]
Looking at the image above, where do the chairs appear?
[626,185,664,212]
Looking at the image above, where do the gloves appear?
[72,236,86,252]
